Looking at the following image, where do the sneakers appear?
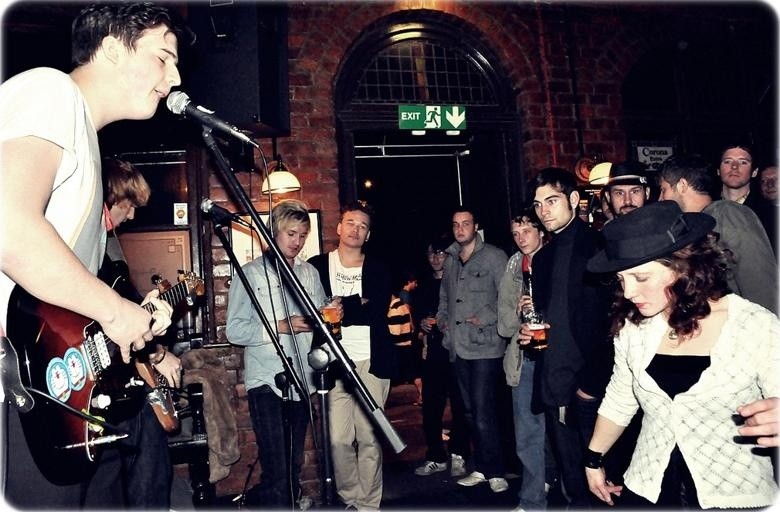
[414,461,447,476]
[449,453,466,478]
[457,471,488,486]
[489,477,509,493]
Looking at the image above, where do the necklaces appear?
[669,328,679,339]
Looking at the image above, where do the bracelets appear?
[153,346,168,366]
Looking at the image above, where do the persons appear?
[600,186,615,226]
[408,238,476,481]
[605,161,653,217]
[226,199,343,512]
[101,158,183,388]
[375,269,427,406]
[497,210,555,510]
[518,166,615,510]
[655,150,778,314]
[308,201,391,510]
[761,163,778,203]
[0,1,183,510]
[435,207,510,493]
[583,200,778,510]
[715,146,777,256]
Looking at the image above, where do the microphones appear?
[199,197,253,230]
[165,89,262,150]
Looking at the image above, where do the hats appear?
[586,200,717,275]
[602,161,650,191]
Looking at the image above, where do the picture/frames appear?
[231,208,323,269]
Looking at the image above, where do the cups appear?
[517,296,535,321]
[523,315,547,348]
[321,297,342,340]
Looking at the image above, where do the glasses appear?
[426,251,447,257]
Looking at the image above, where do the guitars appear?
[135,275,182,437]
[0,272,205,486]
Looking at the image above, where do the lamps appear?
[259,158,303,197]
